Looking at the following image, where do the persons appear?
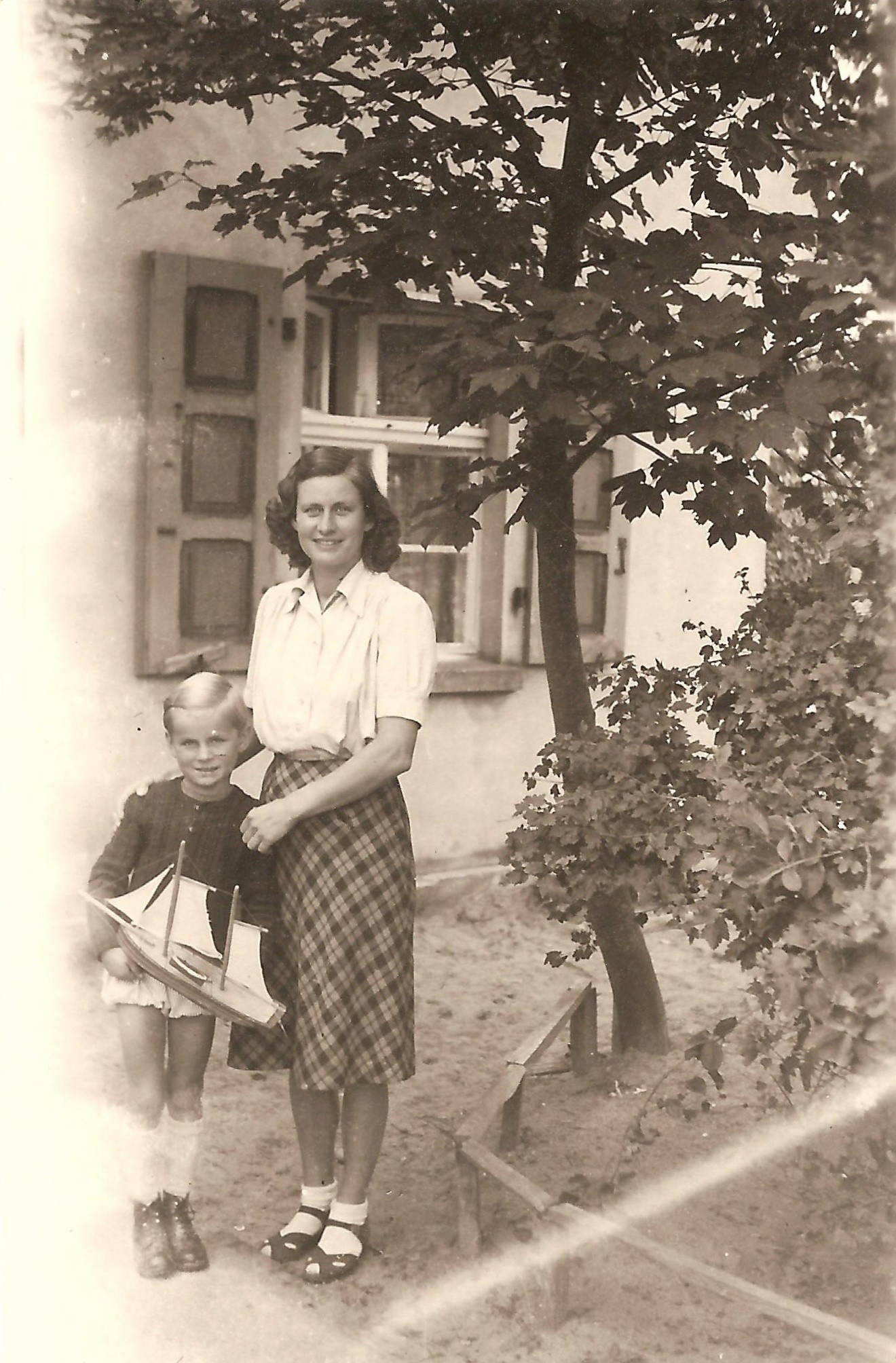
[84,670,279,1279]
[136,447,440,1279]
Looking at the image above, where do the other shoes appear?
[259,1206,330,1261]
[302,1218,370,1284]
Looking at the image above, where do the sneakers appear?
[162,1190,210,1271]
[132,1194,174,1278]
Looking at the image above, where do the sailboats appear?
[77,838,288,1030]
